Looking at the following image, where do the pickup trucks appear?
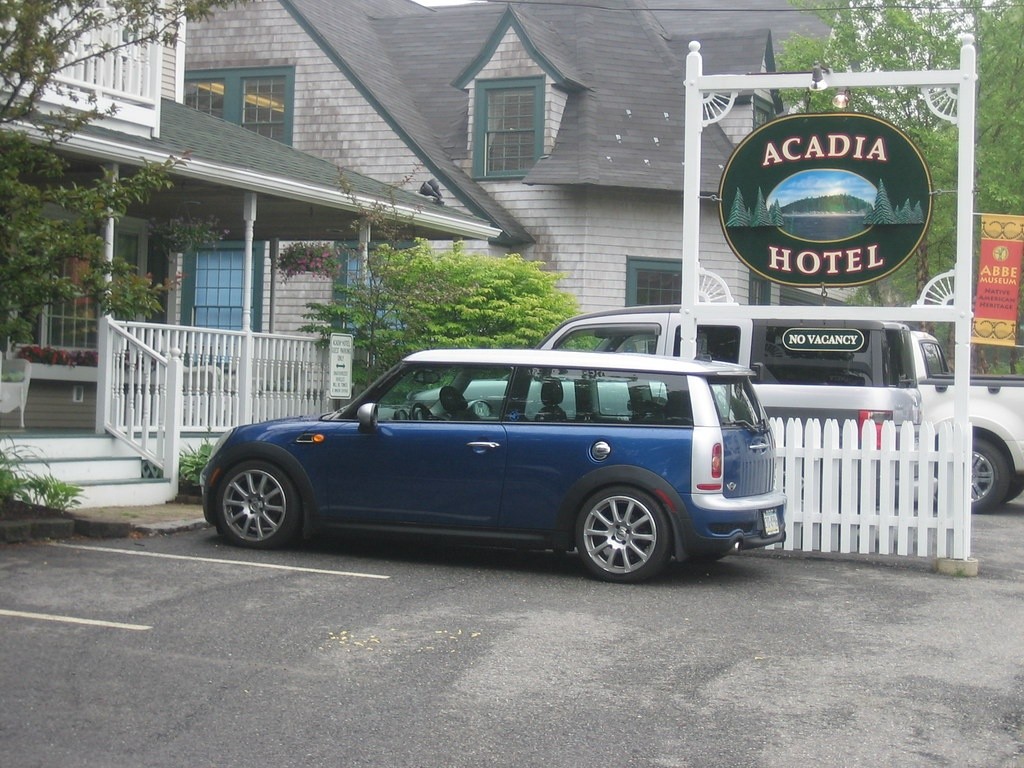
[911,329,1024,514]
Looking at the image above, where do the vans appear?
[199,348,787,583]
[410,303,938,510]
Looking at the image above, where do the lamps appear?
[418,178,444,206]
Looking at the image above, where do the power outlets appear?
[73,385,83,402]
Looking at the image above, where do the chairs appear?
[627,389,690,423]
[439,386,482,420]
[534,378,568,423]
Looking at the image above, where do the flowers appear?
[272,240,342,286]
[144,215,227,263]
[18,346,156,374]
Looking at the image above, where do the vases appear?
[5,336,157,404]
